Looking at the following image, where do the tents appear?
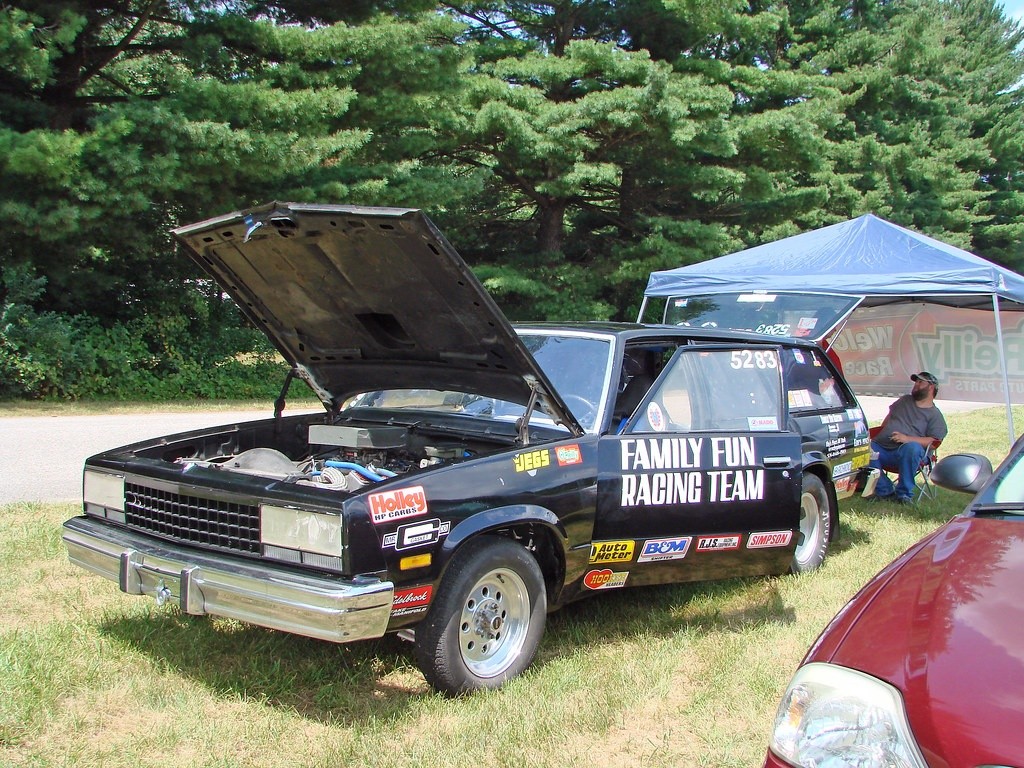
[637,214,1024,446]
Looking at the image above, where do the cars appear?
[758,428,1024,767]
[59,199,872,700]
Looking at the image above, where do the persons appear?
[856,372,948,503]
[811,308,844,379]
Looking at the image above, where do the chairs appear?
[847,427,943,502]
[609,350,659,434]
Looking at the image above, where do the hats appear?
[911,372,938,390]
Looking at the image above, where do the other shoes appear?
[898,494,913,505]
[867,492,895,503]
